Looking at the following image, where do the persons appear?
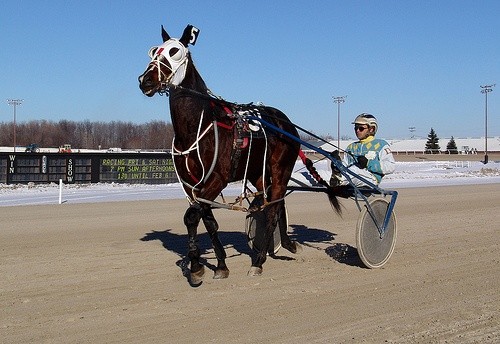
[286,113,396,188]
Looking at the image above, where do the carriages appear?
[138,23,398,287]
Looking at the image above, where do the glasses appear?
[354,126,368,132]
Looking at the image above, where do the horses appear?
[139,24,301,286]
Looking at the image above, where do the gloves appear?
[357,156,368,169]
[332,150,341,161]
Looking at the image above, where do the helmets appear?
[351,113,378,134]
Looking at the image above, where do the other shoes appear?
[329,155,343,188]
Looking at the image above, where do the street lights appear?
[480,84,495,164]
[6,99,25,152]
[332,95,347,153]
